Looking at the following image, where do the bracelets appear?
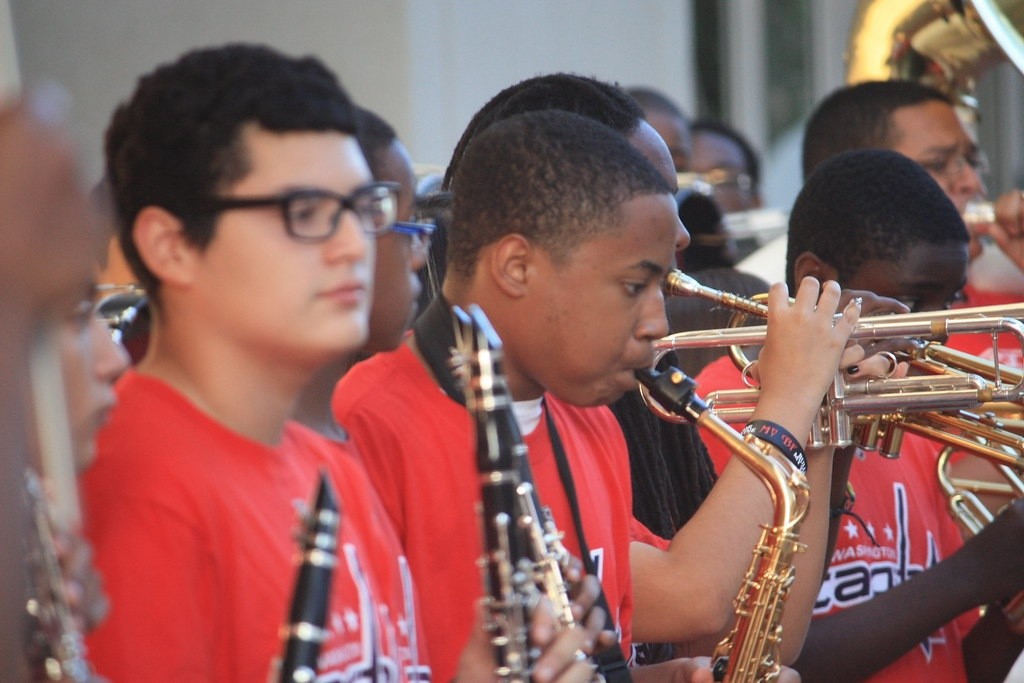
[734,417,813,475]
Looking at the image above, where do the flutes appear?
[445,300,611,683]
[268,465,348,682]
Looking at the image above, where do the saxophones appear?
[635,362,815,683]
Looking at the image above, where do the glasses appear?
[391,218,438,253]
[913,147,992,174]
[886,291,971,313]
[87,280,149,342]
[188,180,404,241]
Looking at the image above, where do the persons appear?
[283,98,622,683]
[18,246,130,475]
[2,90,98,681]
[682,144,1024,683]
[73,41,600,683]
[800,80,1022,455]
[332,112,802,683]
[613,85,699,184]
[661,184,785,386]
[444,71,897,662]
[684,116,760,220]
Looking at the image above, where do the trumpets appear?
[639,191,1024,632]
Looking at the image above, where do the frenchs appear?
[839,2,1024,140]
